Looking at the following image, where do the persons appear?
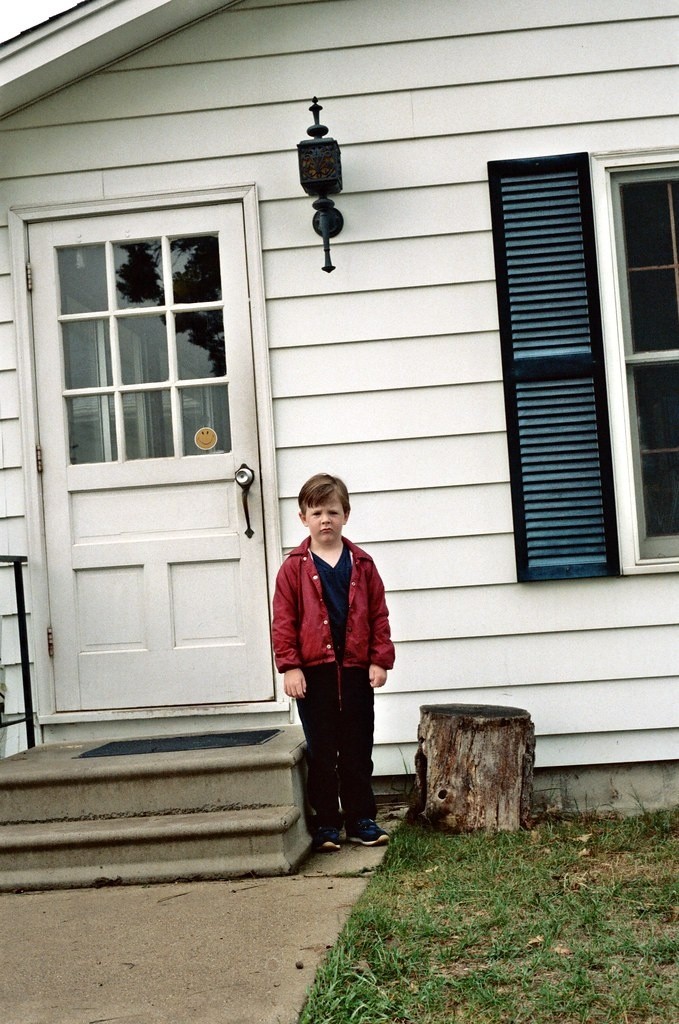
[272,474,395,851]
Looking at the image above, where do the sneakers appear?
[314,825,341,849]
[346,818,390,846]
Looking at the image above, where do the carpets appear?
[71,728,285,758]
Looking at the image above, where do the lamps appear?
[297,96,343,273]
